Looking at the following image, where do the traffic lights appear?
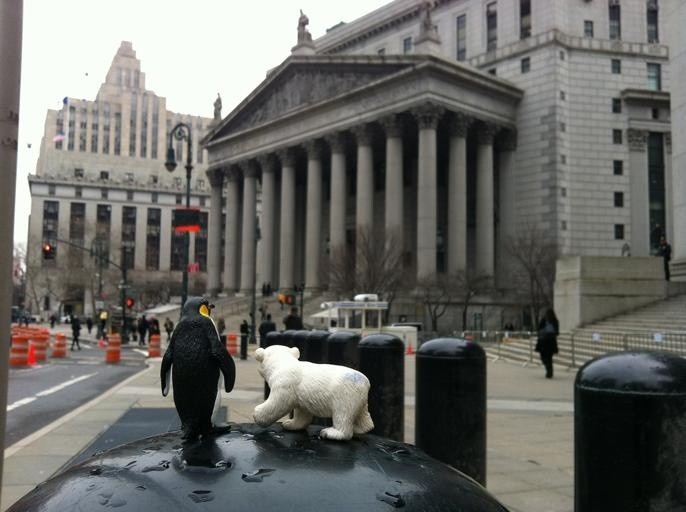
[284,292,297,305]
[42,243,49,259]
[125,297,134,309]
[50,245,56,260]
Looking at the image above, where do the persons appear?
[535,309,559,377]
[50,314,106,351]
[283,307,302,330]
[240,281,275,348]
[130,315,174,346]
[657,236,671,281]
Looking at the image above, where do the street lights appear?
[164,122,193,316]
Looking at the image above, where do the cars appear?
[59,314,76,324]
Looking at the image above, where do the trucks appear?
[11,306,36,323]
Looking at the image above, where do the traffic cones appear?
[405,340,414,355]
[26,342,37,365]
[98,336,105,347]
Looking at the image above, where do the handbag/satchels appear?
[538,325,555,339]
